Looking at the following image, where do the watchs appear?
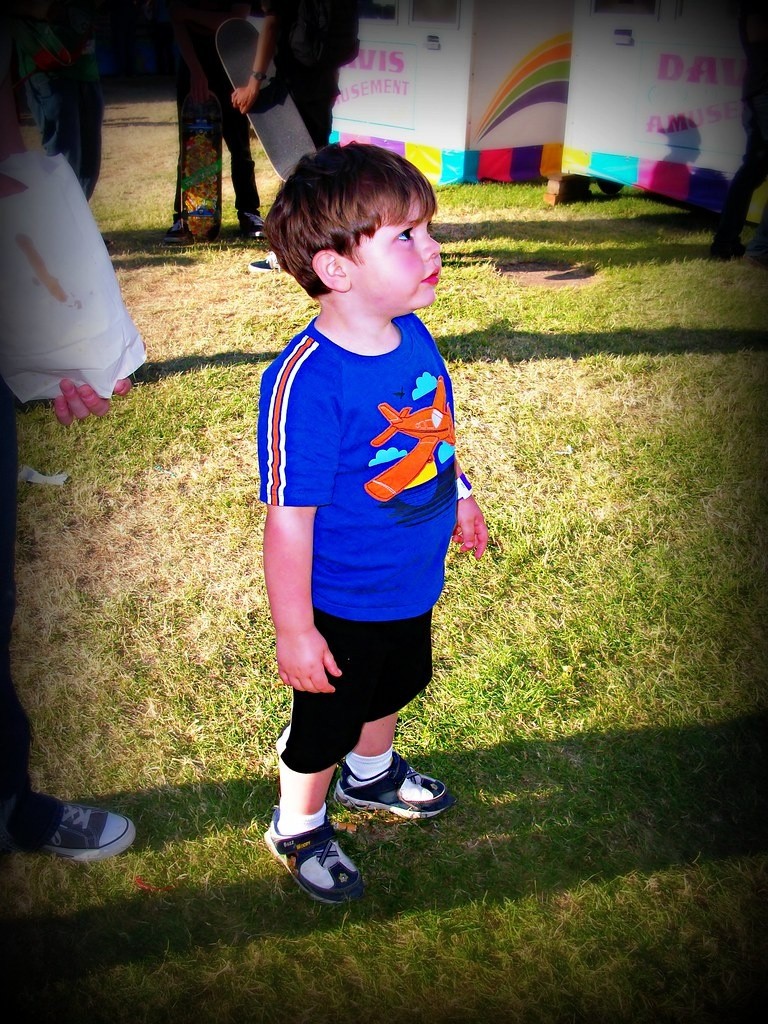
[248,69,267,81]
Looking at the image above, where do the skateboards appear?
[214,17,316,183]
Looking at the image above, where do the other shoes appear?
[711,242,746,259]
[743,253,768,271]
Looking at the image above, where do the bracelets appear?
[457,473,473,501]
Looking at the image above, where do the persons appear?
[711,0,768,269]
[133,0,357,242]
[259,142,488,902]
[0,0,136,861]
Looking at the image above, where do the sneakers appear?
[44,797,137,862]
[333,751,452,819]
[262,808,365,903]
[238,210,266,238]
[247,251,281,278]
[164,220,192,242]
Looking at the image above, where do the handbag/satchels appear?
[0,151,149,405]
